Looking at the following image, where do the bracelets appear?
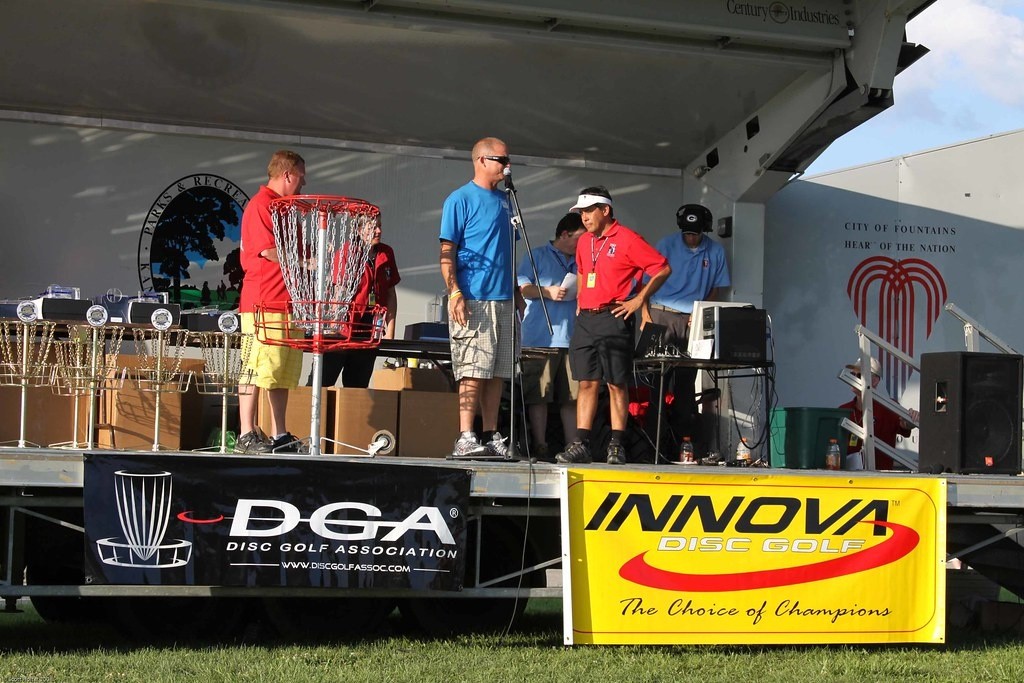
[449,289,462,300]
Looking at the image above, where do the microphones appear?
[503,167,512,194]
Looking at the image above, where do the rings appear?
[624,309,626,313]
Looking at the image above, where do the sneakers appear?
[555,438,592,463]
[484,432,521,462]
[452,432,489,457]
[606,438,625,464]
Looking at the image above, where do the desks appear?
[632,357,776,467]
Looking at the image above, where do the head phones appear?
[676,204,713,233]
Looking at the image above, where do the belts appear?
[651,303,687,315]
[588,305,620,315]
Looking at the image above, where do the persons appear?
[306,208,402,388]
[438,138,528,456]
[639,205,732,464]
[235,151,332,454]
[556,186,673,465]
[839,356,920,470]
[518,212,587,462]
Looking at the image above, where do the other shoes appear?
[534,443,557,463]
[270,432,306,453]
[234,430,271,455]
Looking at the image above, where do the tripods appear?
[446,190,554,464]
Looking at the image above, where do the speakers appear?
[920,352,1023,475]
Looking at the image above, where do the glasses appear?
[478,156,509,165]
[849,371,861,378]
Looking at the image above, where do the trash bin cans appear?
[768,406,853,470]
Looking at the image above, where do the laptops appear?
[634,322,668,356]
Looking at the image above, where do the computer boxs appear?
[702,307,767,363]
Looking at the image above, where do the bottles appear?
[736,438,751,463]
[372,312,383,340]
[679,437,694,463]
[826,438,840,471]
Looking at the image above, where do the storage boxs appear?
[767,406,852,470]
[0,341,209,450]
[254,364,463,459]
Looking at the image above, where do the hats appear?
[569,195,612,212]
[846,356,882,379]
[681,208,705,235]
